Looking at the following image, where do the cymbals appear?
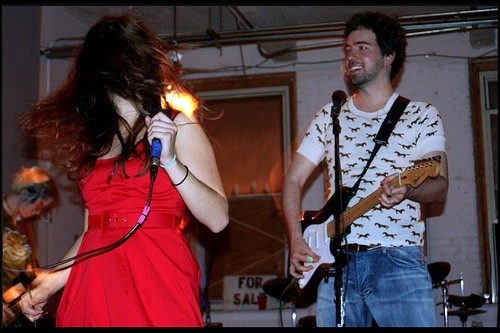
[263,278,318,305]
[433,279,461,288]
[440,310,486,315]
[427,262,451,284]
[451,294,486,307]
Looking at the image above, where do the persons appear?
[2,166,57,328]
[13,13,229,327]
[282,12,448,328]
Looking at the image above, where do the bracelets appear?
[160,152,177,168]
[172,165,189,186]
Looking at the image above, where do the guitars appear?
[296,154,442,290]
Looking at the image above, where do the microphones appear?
[331,89,347,119]
[150,110,167,166]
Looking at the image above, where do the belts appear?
[341,244,371,252]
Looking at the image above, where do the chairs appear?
[262,262,451,327]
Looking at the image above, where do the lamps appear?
[168,6,183,69]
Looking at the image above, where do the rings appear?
[306,256,313,263]
